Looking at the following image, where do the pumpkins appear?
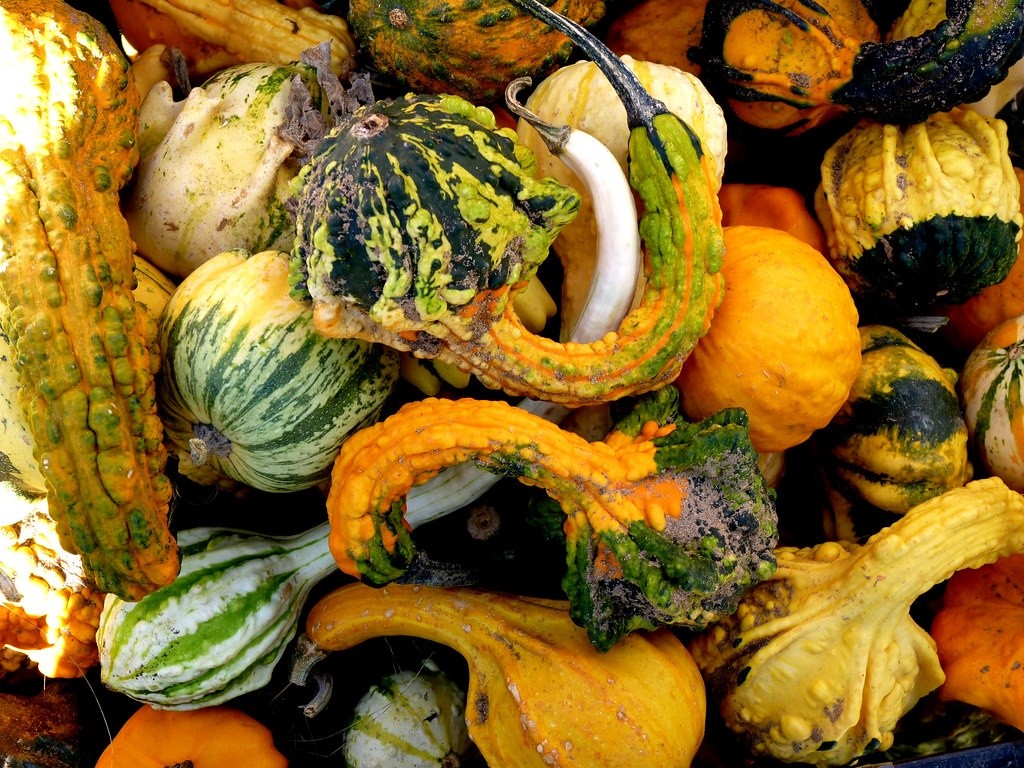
[1,1,1024,768]
